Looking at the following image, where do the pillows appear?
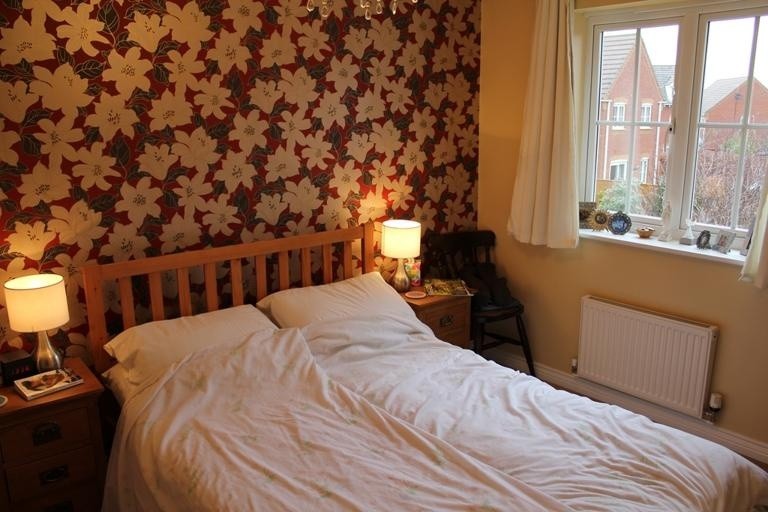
[102,303,280,364]
[256,270,417,332]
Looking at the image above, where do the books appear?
[10,365,85,401]
[423,278,475,298]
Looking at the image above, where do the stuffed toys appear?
[460,259,512,308]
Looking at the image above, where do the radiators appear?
[575,294,717,422]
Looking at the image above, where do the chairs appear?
[435,229,534,376]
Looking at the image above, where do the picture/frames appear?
[739,213,755,255]
[712,228,735,254]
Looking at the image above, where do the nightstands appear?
[397,277,476,350]
[1,355,105,512]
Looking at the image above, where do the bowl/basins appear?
[636,228,655,238]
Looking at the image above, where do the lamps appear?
[380,219,421,292]
[3,274,73,371]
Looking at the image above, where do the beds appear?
[84,222,766,510]
